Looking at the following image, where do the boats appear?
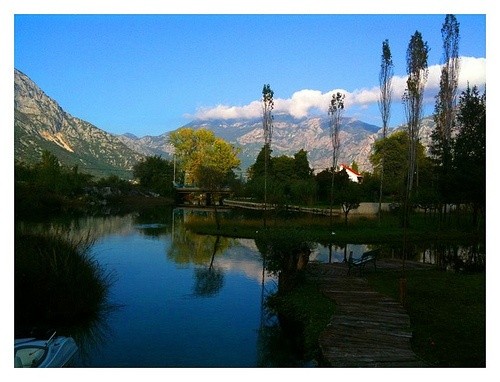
[15,336,80,368]
